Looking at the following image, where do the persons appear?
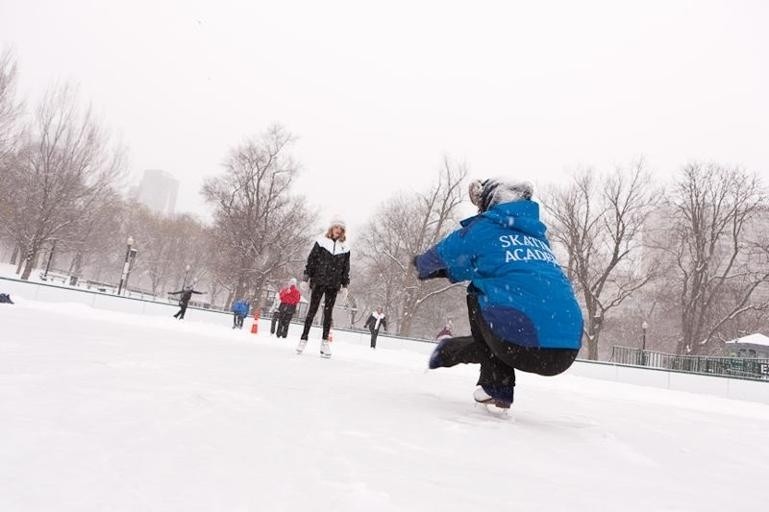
[414,178,584,411]
[231,278,301,339]
[295,225,350,358]
[363,305,387,349]
[168,285,208,320]
[436,324,453,341]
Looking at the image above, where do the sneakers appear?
[296,339,306,352]
[370,345,376,349]
[429,335,452,369]
[320,339,331,355]
[473,386,512,408]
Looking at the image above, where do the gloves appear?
[364,325,366,328]
[286,289,292,296]
[339,287,348,301]
[301,281,309,291]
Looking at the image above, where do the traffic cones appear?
[250,310,260,335]
[327,324,334,352]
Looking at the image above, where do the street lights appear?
[180,263,192,306]
[116,235,134,295]
[641,320,649,366]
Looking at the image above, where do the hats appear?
[329,213,346,230]
[469,178,533,208]
[287,277,297,287]
